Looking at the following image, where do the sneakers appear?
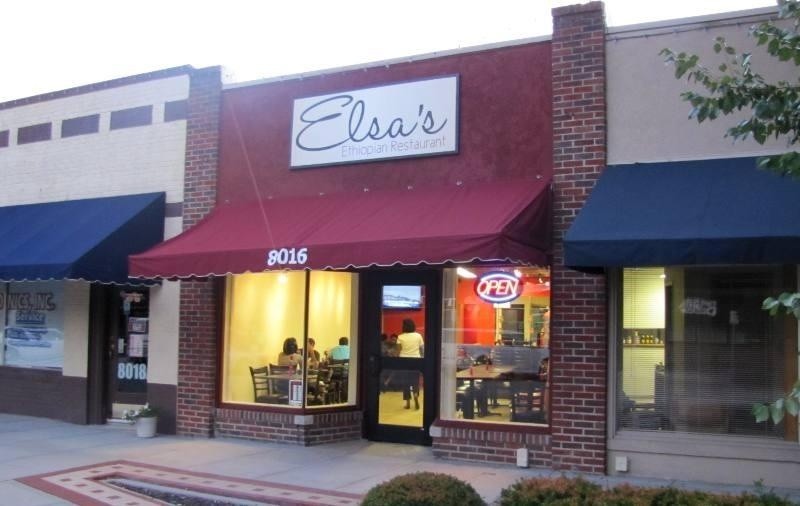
[404,397,420,410]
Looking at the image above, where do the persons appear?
[277,332,398,395]
[396,317,424,410]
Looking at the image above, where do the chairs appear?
[248,358,350,406]
[508,376,547,422]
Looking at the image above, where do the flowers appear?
[121,401,159,425]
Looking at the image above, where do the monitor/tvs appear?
[381,284,423,311]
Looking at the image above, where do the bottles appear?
[623,330,657,345]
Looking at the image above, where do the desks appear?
[456,362,513,417]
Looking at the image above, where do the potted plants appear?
[135,414,158,439]
